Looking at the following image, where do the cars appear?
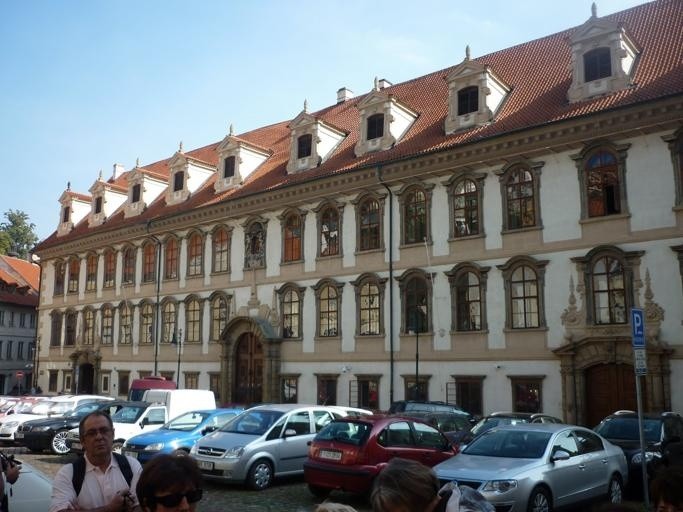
[189,404,358,490]
[301,415,459,502]
[464,412,565,453]
[0,391,122,456]
[313,405,374,430]
[428,422,628,512]
[401,410,472,444]
[119,407,245,471]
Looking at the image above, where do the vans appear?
[387,400,470,421]
[124,376,176,403]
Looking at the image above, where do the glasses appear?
[82,427,114,437]
[151,488,202,507]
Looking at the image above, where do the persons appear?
[369,457,496,512]
[135,454,204,512]
[0,453,22,512]
[51,412,142,512]
[316,501,358,512]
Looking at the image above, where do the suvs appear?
[592,408,682,499]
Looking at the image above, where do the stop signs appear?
[16,371,24,380]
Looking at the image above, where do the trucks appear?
[64,387,217,458]
